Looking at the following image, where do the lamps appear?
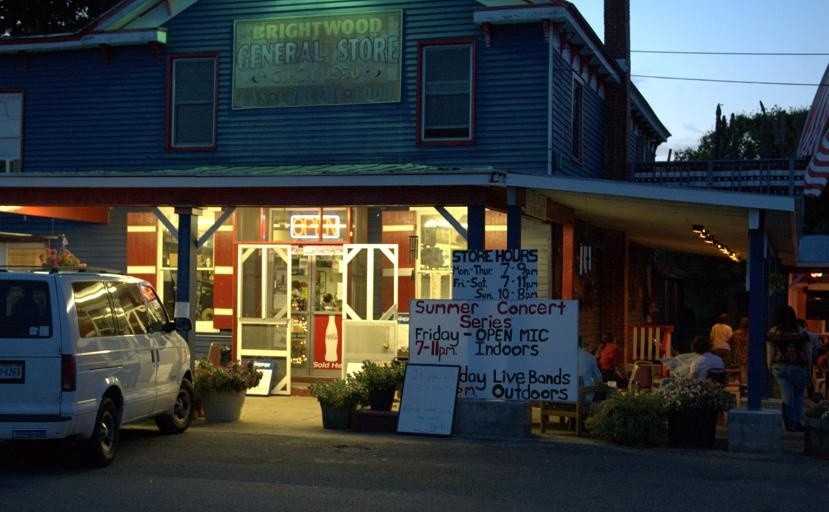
[687,225,722,248]
[578,234,593,276]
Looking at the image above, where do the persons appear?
[292,280,307,312]
[577,296,829,433]
[323,294,334,308]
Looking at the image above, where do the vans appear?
[2,264,196,470]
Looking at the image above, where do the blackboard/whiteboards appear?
[396,363,462,438]
[408,249,580,404]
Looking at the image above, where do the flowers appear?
[191,358,263,400]
[806,400,829,424]
[39,248,87,272]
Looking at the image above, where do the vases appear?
[802,411,829,459]
[667,409,718,447]
[200,389,247,423]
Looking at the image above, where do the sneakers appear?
[790,422,806,432]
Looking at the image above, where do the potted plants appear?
[584,390,664,446]
[307,360,404,429]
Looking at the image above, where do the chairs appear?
[540,360,742,433]
